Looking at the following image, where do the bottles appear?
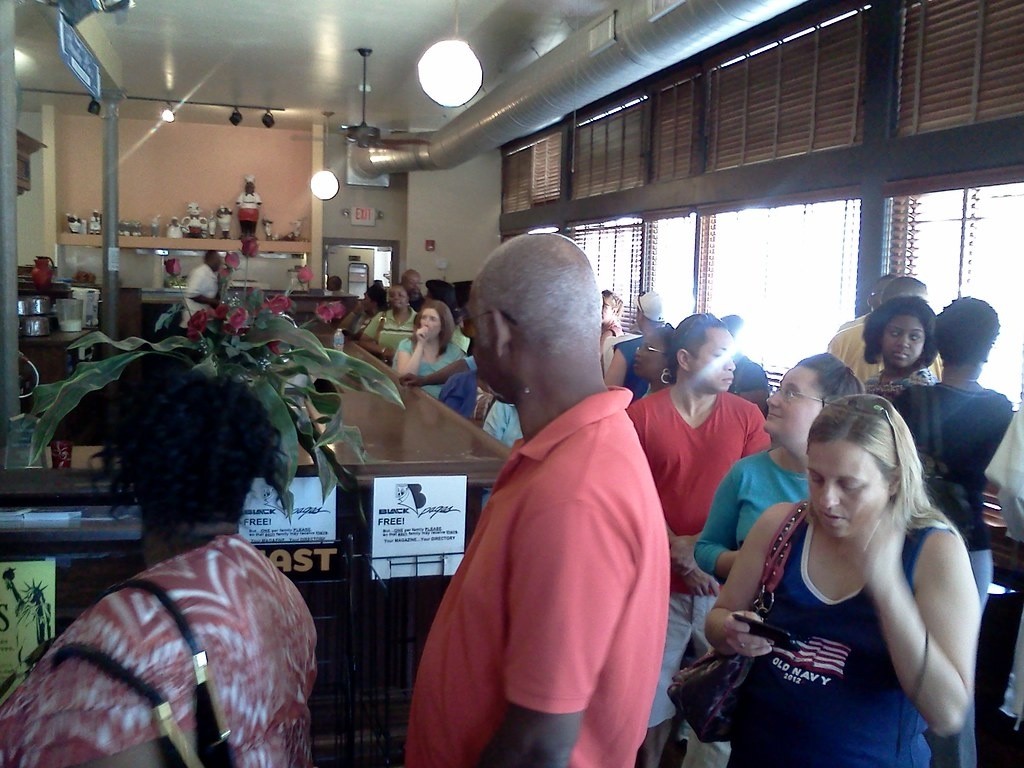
[333,328,344,353]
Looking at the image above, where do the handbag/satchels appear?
[667,605,770,743]
[49,578,234,768]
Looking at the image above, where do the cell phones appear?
[733,613,801,652]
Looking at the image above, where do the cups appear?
[51,440,73,469]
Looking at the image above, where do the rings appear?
[740,643,744,648]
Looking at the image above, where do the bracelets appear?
[382,347,387,354]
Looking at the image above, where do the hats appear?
[634,291,663,322]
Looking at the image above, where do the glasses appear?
[601,290,617,306]
[637,291,646,314]
[458,307,518,339]
[680,313,716,344]
[770,384,823,403]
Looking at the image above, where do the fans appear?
[287,49,432,158]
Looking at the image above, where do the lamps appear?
[261,106,275,129]
[419,2,483,108]
[87,94,100,115]
[310,109,341,199]
[229,107,242,126]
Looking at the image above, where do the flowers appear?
[22,239,386,531]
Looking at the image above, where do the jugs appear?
[32,256,55,287]
[56,299,83,332]
[19,293,51,336]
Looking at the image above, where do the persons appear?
[0,368,318,768]
[178,250,223,361]
[406,232,669,768]
[702,395,980,768]
[600,274,1024,768]
[340,270,523,506]
